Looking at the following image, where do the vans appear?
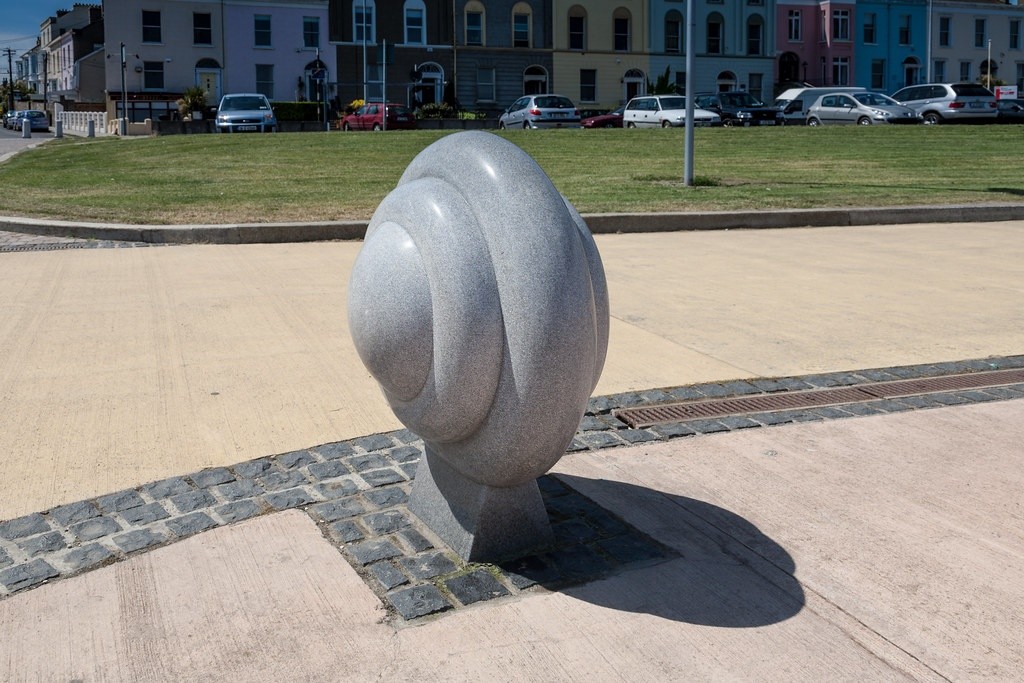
[774,87,865,125]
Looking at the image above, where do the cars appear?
[211,93,278,133]
[499,94,581,128]
[3,110,49,132]
[581,107,626,129]
[806,92,923,126]
[995,99,1024,124]
[338,102,417,131]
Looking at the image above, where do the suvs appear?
[693,91,785,126]
[623,95,722,128]
[891,83,1000,125]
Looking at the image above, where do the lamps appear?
[165,59,171,63]
[296,48,302,53]
[617,58,620,65]
